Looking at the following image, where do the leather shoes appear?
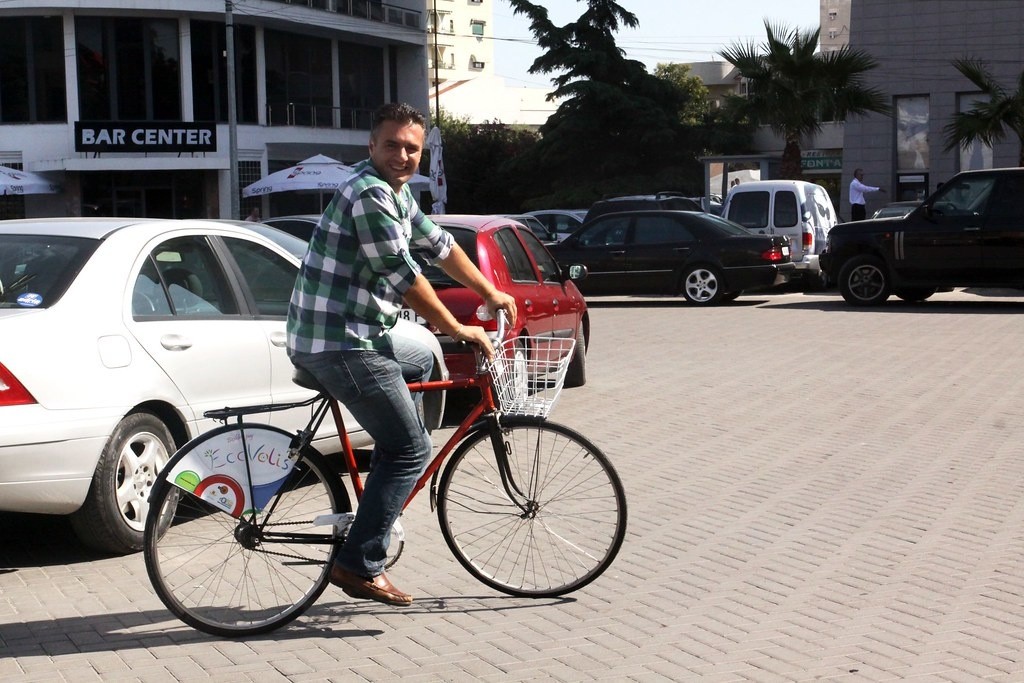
[328,556,411,607]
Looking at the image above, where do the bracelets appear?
[450,324,464,342]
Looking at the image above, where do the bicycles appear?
[142,312,628,632]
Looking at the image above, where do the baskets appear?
[486,334,572,420]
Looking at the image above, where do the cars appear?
[490,215,561,245]
[871,201,969,220]
[524,210,588,242]
[233,214,322,269]
[541,209,796,306]
[0,216,450,552]
[397,214,590,411]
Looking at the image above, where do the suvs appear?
[581,192,705,245]
[818,165,1024,307]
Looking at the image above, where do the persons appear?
[288,103,519,606]
[850,168,881,222]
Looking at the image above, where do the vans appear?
[719,180,839,287]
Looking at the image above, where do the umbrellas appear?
[242,151,440,214]
[0,162,62,196]
[428,125,448,214]
